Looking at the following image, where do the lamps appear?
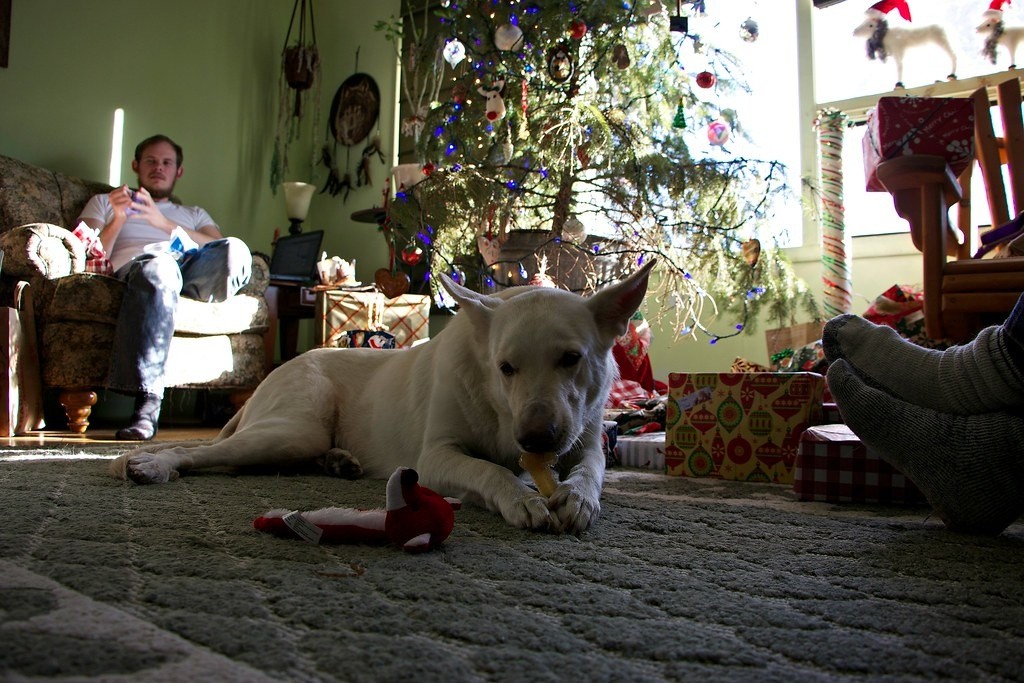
[284,180,316,233]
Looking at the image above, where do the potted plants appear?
[373,1,821,344]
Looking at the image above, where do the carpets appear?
[1,438,1024,683]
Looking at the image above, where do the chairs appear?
[915,77,1024,340]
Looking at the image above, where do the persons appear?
[821,293,1024,535]
[79,133,253,440]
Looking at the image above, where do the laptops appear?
[270,230,324,283]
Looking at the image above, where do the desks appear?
[267,281,318,364]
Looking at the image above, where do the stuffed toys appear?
[254,465,462,554]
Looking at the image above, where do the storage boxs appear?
[316,289,431,349]
[862,95,976,192]
[616,321,917,508]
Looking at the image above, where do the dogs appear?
[104,257,658,531]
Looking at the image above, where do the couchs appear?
[0,155,270,435]
